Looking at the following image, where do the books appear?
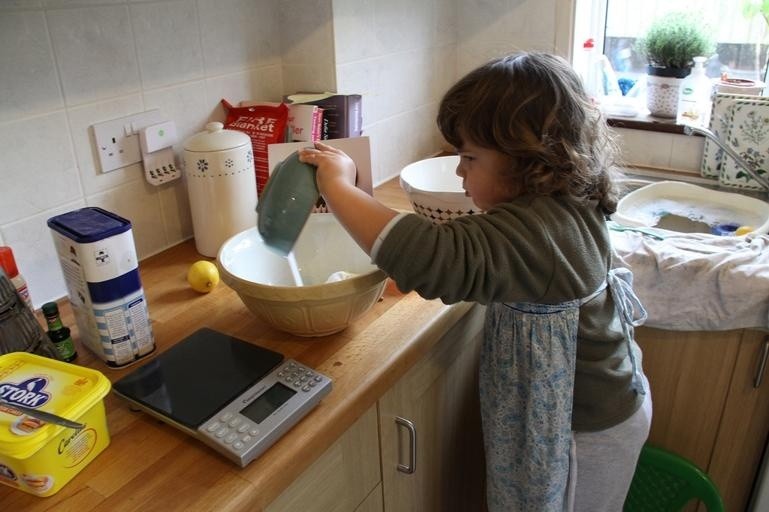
[239,90,374,145]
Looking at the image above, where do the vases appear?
[715,77,766,94]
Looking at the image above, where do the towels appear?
[608,221,769,331]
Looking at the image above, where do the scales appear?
[111,328,334,468]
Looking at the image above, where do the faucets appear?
[684,124,769,193]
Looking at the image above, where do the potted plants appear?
[640,11,714,118]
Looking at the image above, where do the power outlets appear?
[90,109,165,176]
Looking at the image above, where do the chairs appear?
[624,444,725,511]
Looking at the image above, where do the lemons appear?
[188,260,220,293]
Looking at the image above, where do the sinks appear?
[613,174,769,248]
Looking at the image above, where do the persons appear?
[299,50,652,512]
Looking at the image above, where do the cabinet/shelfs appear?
[379,307,484,512]
[624,328,769,510]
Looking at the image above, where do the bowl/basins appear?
[400,155,488,226]
[254,151,319,258]
[216,213,390,338]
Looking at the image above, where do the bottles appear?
[0,245,38,319]
[41,302,79,363]
